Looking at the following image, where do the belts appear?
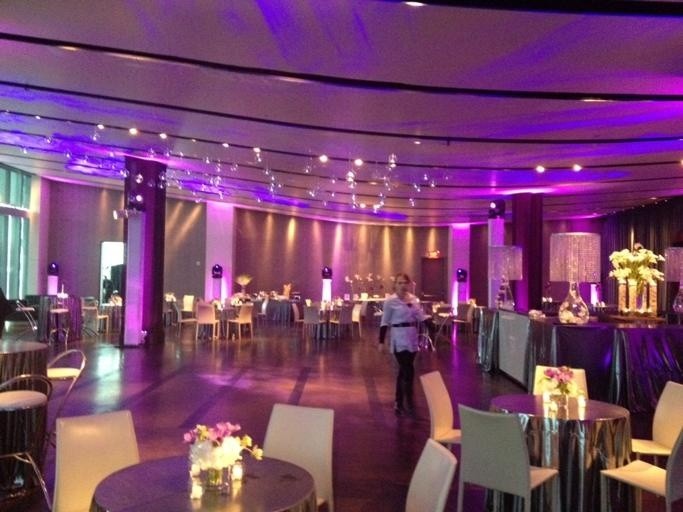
[392,322,417,327]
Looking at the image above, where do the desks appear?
[0,339,49,500]
[89,453,317,512]
[484,394,631,512]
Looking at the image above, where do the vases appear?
[556,390,570,407]
[203,468,227,490]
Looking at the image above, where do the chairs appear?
[407,437,458,512]
[532,364,588,398]
[39,350,86,473]
[629,380,683,512]
[51,410,140,511]
[419,370,461,452]
[262,403,335,512]
[0,373,52,511]
[457,402,559,512]
[599,429,683,512]
[0,288,37,341]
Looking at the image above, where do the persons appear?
[377,273,434,418]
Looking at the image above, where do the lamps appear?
[664,247,683,313]
[488,244,523,310]
[547,231,601,325]
[112,205,142,221]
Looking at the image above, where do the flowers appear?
[542,366,575,393]
[184,420,263,474]
[608,248,666,296]
